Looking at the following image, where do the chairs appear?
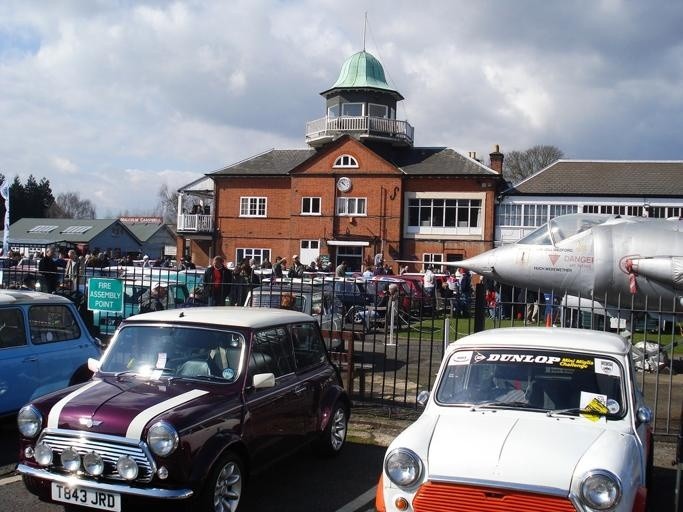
[161,348,273,384]
[497,362,599,411]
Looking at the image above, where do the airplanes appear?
[441,214,682,316]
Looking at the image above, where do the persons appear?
[0,241,545,348]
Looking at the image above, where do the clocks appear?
[336,176,353,193]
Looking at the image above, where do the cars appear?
[15,306,353,511]
[374,323,656,512]
[1,287,100,423]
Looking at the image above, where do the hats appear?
[151,282,168,290]
